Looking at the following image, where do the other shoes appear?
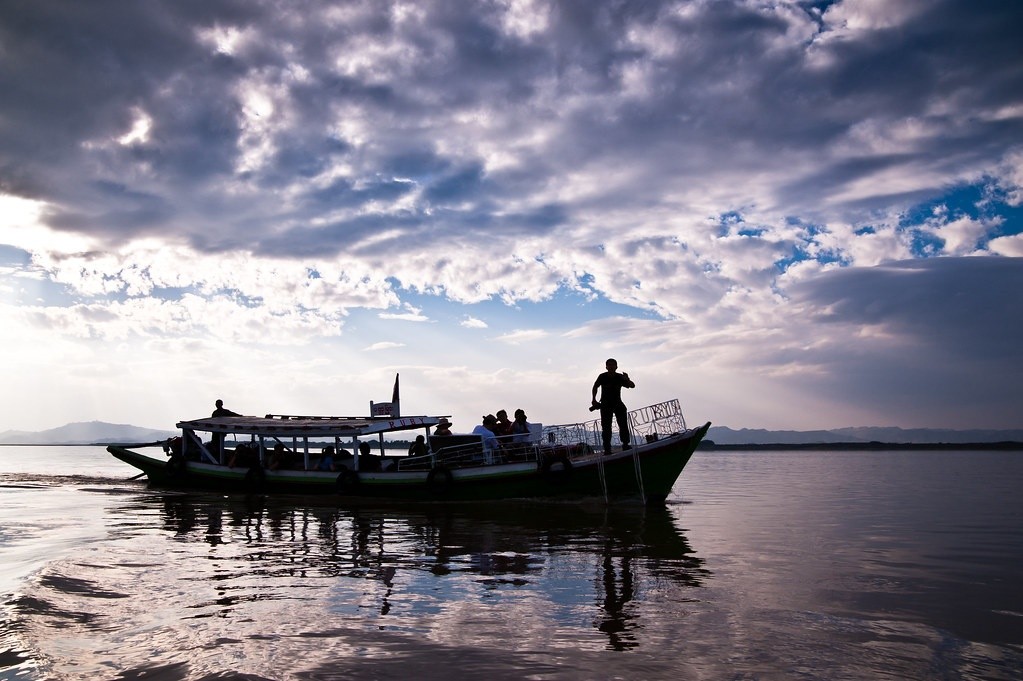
[623,445,632,451]
[604,450,611,455]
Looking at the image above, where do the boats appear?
[104,373,713,514]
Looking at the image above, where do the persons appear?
[319,445,337,471]
[472,414,499,449]
[163,427,203,463]
[269,444,292,470]
[230,443,258,466]
[591,358,635,456]
[495,410,512,435]
[212,400,243,445]
[409,435,428,456]
[509,409,530,433]
[358,442,381,473]
[434,419,453,435]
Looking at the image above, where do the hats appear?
[436,419,452,428]
[483,415,499,426]
